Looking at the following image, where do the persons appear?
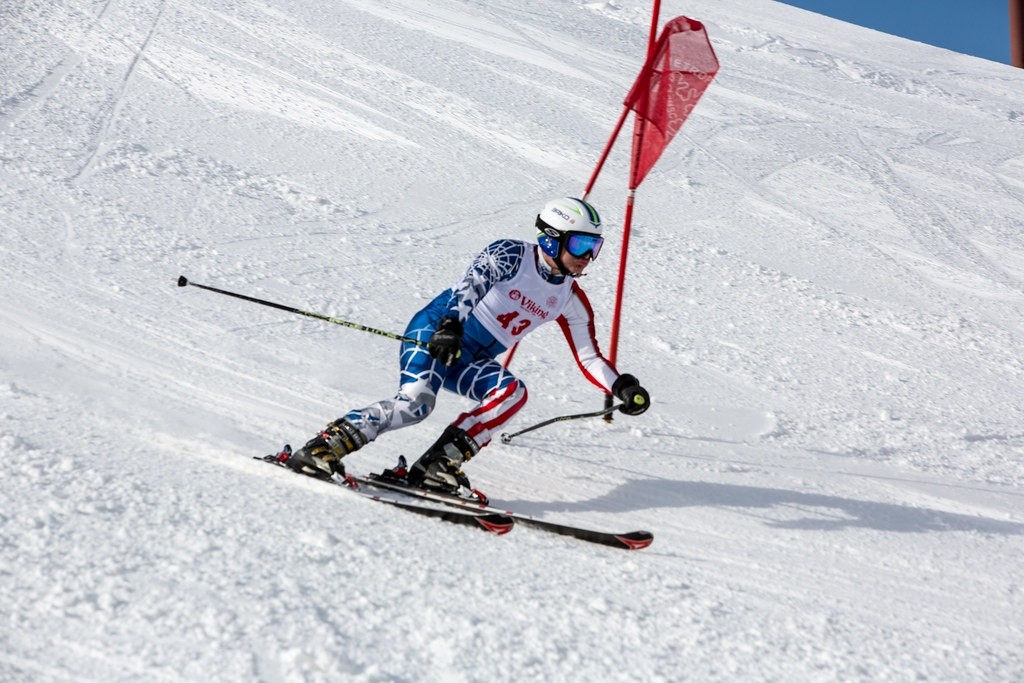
[292,197,650,503]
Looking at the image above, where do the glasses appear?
[563,229,604,262]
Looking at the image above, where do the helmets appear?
[536,199,602,258]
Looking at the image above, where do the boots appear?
[408,425,480,496]
[291,418,370,476]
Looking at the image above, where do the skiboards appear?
[252,448,656,552]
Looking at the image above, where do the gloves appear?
[428,315,463,367]
[611,373,650,415]
